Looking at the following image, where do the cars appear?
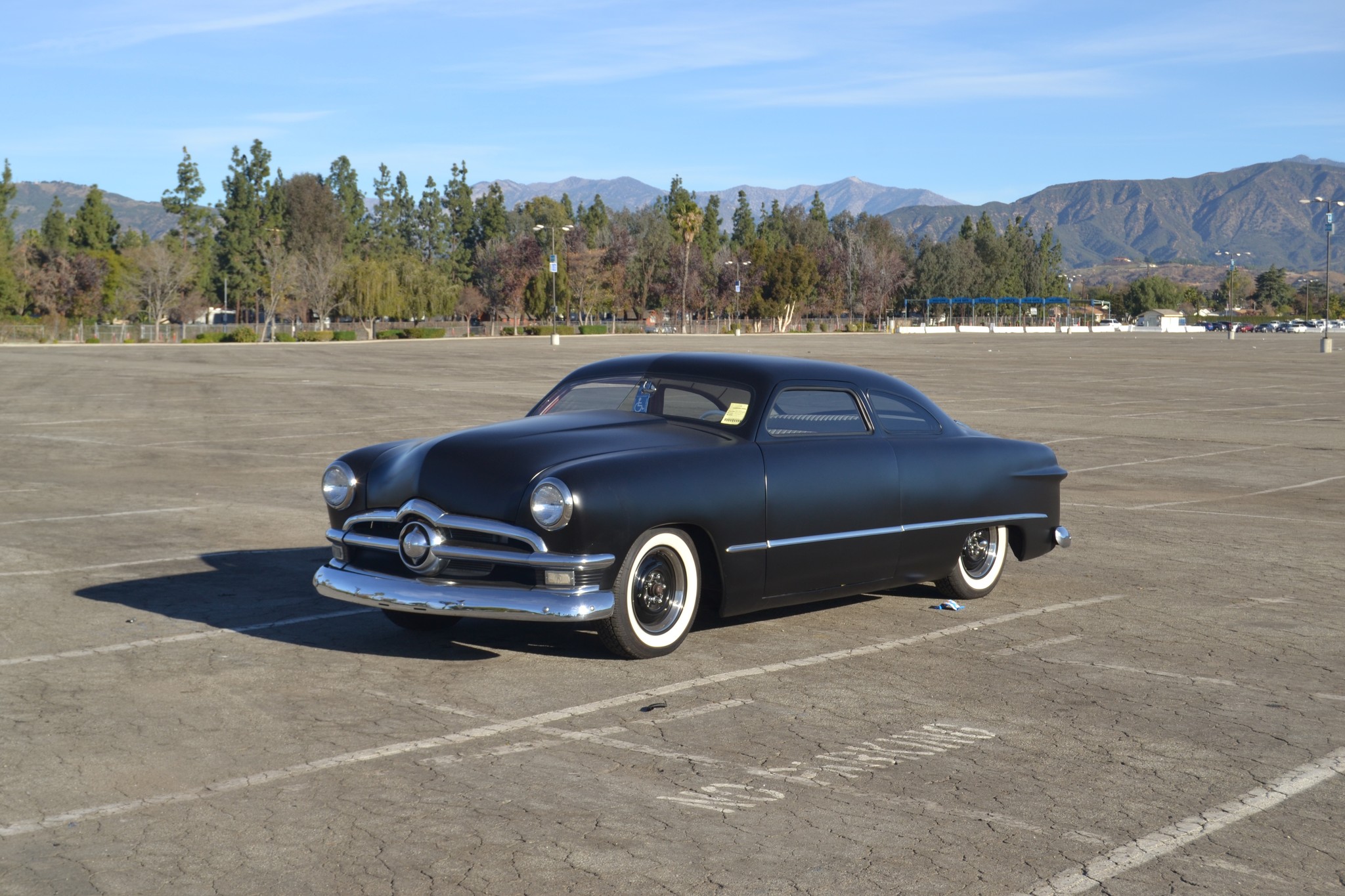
[1099,319,1122,326]
[1193,317,1345,333]
[312,349,1072,660]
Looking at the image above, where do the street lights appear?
[1058,274,1082,329]
[1298,196,1345,339]
[532,223,574,334]
[1298,279,1318,322]
[724,261,751,329]
[1214,251,1250,331]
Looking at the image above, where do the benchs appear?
[765,413,864,437]
